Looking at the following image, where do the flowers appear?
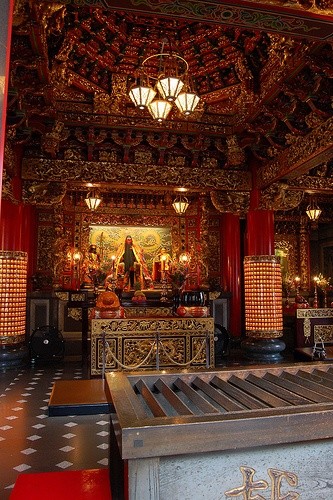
[80,257,114,284]
[316,273,333,292]
[282,272,297,293]
[166,261,190,292]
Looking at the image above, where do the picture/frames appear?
[320,241,333,285]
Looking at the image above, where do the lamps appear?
[172,192,190,215]
[84,188,104,212]
[129,33,201,124]
[306,195,322,221]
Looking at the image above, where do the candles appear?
[295,277,299,287]
[162,255,166,270]
[112,255,115,269]
[314,276,317,291]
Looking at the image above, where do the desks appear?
[89,306,215,374]
[296,308,333,348]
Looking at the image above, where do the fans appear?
[215,324,230,357]
[27,325,66,367]
